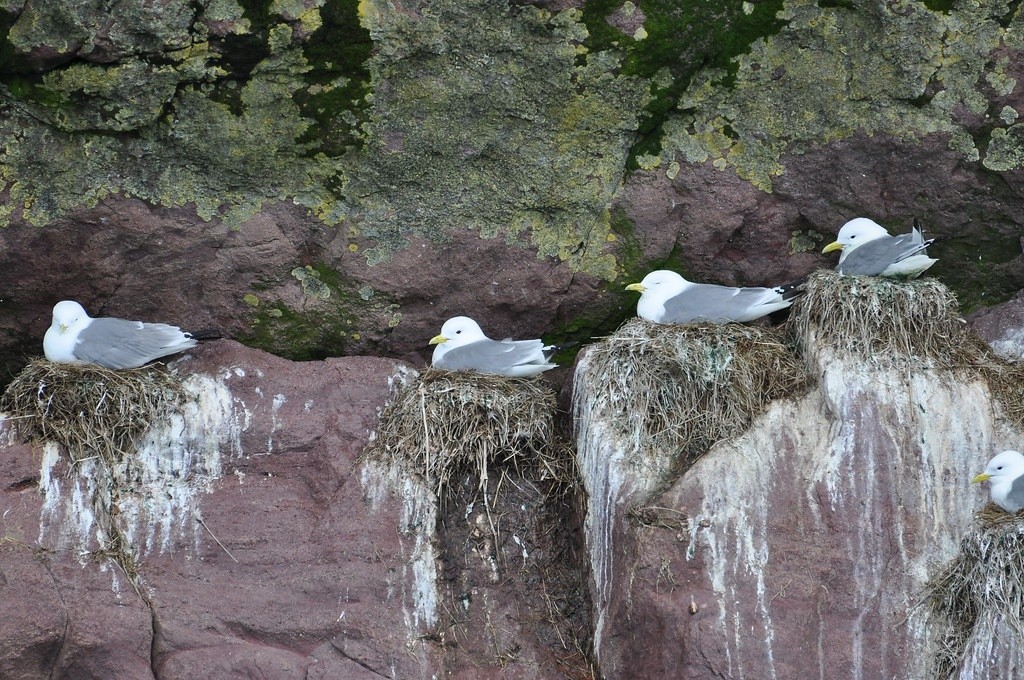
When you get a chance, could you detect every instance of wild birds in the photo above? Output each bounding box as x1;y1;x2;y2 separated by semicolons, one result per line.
41;298;205;379
425;315;562;382
969;448;1023;518
624;266;813;333
822;214;945;280
